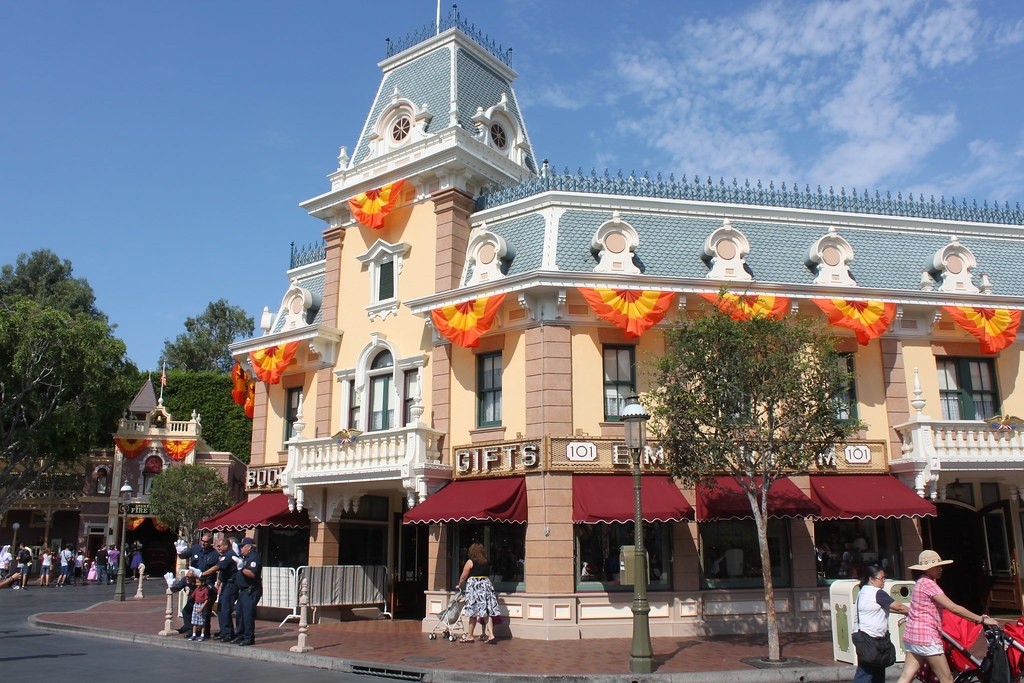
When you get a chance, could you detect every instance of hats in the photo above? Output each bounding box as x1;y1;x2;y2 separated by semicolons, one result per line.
908;550;954;571
238;537;255;546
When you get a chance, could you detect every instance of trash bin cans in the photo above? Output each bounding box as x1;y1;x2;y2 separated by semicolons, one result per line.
829;578;917;665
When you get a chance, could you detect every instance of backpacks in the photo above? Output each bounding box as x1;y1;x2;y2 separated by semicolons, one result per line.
20;550;31;564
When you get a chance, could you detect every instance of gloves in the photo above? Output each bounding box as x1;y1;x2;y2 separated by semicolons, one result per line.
164;572;176;588
11;572;21;581
232;556;244;571
188;565;202;578
174;540;188;555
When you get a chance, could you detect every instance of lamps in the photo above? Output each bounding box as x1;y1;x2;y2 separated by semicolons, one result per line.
516;432;523;439
575;428;589;436
109;528;113;535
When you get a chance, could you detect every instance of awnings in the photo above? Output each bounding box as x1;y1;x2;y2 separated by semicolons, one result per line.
809;474;938;522
572;475;696;526
403;477;528;526
198;493;310;534
696;475;821;522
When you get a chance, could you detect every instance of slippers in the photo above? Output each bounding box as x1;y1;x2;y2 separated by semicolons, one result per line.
484;639;497;644
459;638;474;643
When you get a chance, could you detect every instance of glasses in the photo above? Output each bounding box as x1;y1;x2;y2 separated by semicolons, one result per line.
873;576;885;581
200;538;209;543
217;545;221;548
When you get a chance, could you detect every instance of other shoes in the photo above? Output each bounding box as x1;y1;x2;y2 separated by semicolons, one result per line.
183;632;189;638
131;576;135;580
198;637;204;642
213;632;226;640
175;628;184;633
46;583;49;586
40;582;43;586
60;583;64;587
478;634;488;639
20;586;24;590
56;583;59;588
188;636;196;641
239;639;254;646
230;638;243;644
220;635;235;642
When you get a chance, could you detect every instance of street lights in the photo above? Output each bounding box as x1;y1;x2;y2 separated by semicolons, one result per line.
619;390;656;673
113;480;134;601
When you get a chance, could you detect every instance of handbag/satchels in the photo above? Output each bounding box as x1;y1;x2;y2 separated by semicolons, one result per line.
979;630;1014;683
67;561;75;568
50;565;54;571
851;631;896;669
837;562;849;576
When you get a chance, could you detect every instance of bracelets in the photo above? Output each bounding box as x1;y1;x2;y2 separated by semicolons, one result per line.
978;615;984;624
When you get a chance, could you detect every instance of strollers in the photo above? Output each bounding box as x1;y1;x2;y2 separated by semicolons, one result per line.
976;618;1024;683
916;609;985;683
428;587;468;642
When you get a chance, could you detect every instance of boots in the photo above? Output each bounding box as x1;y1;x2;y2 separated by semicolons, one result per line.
77;578;80;586
73;577;77;586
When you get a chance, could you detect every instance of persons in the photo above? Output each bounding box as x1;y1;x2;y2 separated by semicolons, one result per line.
164;533;263;646
456;543;501;644
0;540;143;590
817;542;865;578
851;566;910;683
896;550;999;683
